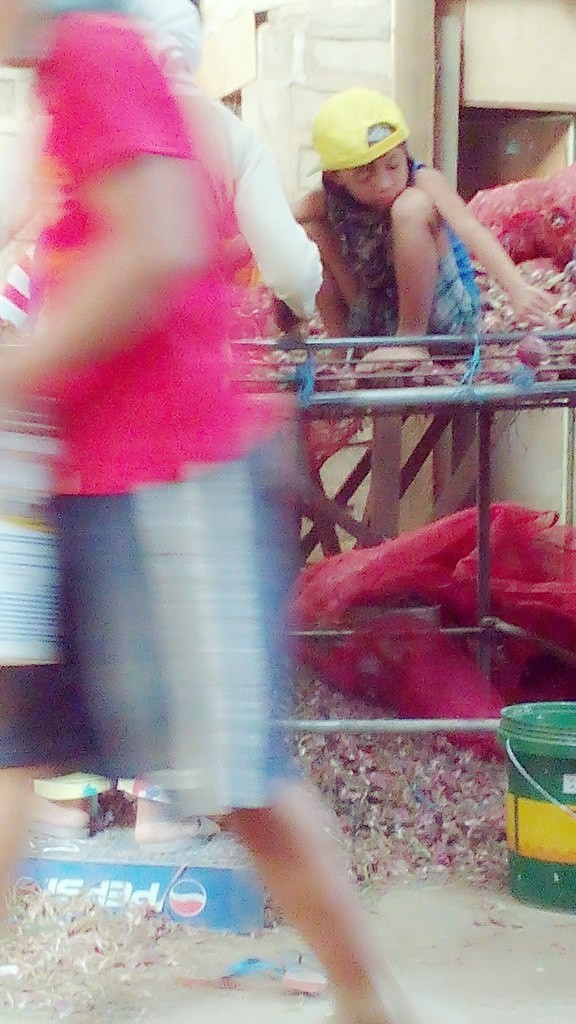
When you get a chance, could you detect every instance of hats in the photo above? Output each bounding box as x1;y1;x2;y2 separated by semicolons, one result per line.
311;88;409;170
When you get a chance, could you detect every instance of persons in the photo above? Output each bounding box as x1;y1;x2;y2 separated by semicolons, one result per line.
3;0;423;1021
291;90;557;361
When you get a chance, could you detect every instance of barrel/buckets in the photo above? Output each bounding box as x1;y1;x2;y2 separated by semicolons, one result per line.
496;702;576;916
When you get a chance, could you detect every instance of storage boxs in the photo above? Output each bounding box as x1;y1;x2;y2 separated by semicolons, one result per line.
17;825;267;930
